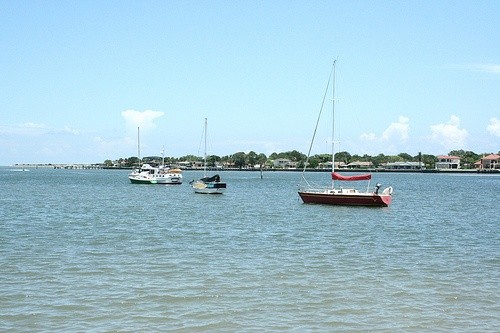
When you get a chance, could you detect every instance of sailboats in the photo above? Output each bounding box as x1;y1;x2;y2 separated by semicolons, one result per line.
128;126;183;185
191;117;227;194
298;57;395;208
